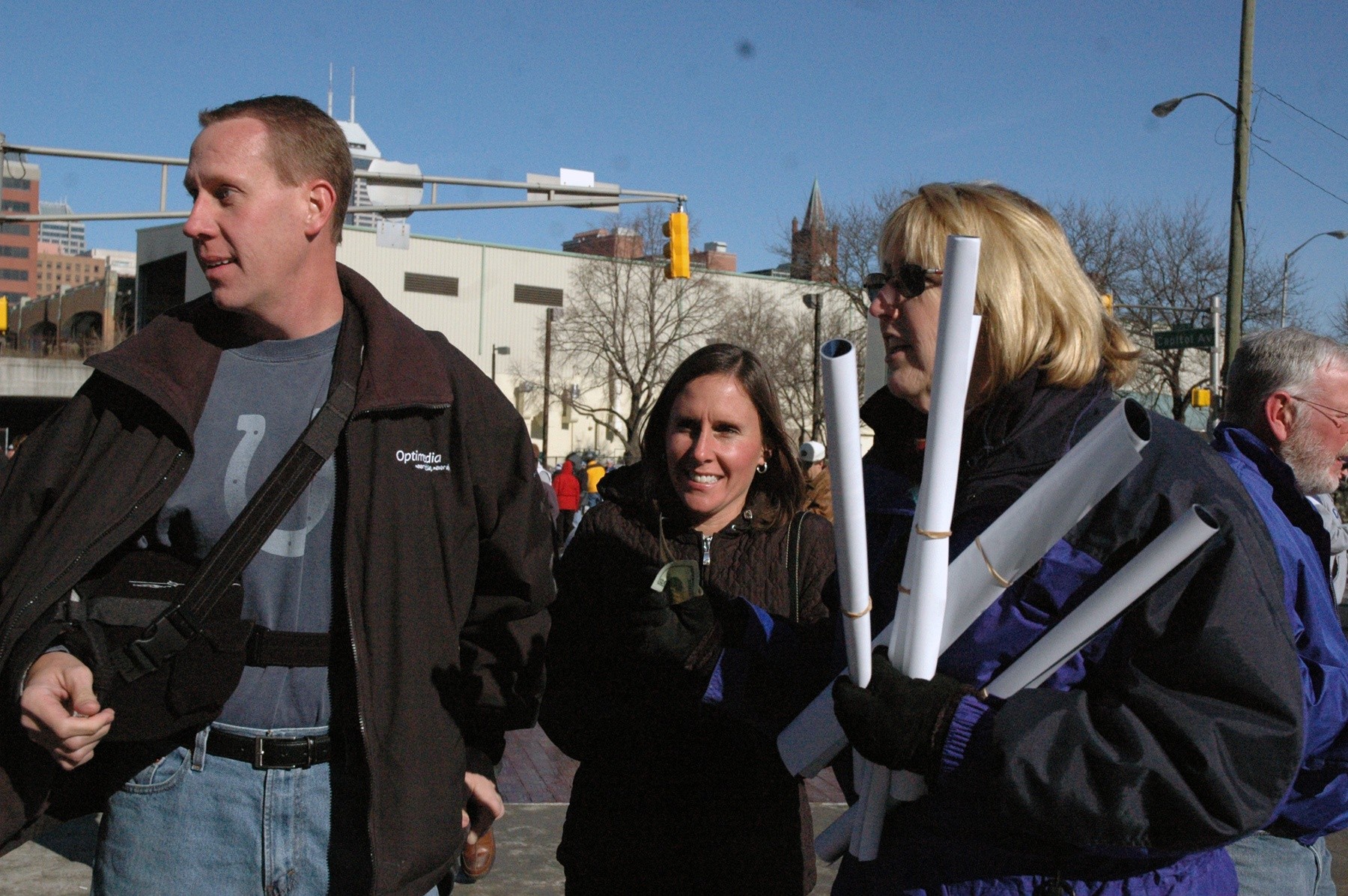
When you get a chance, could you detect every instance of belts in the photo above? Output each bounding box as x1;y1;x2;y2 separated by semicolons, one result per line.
181;725;367;771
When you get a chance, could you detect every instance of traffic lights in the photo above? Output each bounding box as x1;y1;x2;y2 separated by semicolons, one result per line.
661;212;690;280
1099;293;1114;316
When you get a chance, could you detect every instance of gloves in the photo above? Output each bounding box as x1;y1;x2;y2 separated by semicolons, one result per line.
831;645;963;776
620;565;723;677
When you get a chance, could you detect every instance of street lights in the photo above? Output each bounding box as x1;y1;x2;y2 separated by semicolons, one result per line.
1149;90;1252;395
492;343;511;382
1280;225;1348;328
802;292;823;439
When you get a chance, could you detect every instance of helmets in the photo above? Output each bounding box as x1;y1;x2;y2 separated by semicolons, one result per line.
583;451;595;462
799;441;828;463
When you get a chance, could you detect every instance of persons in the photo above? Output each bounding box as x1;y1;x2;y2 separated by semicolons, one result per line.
0;94;557;896
532;442;618;559
539;342;849;896
1205;327;1348;894
799;440;836;526
821;182;1311;895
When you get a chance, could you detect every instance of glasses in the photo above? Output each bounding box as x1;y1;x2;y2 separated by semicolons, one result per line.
863;263;943;305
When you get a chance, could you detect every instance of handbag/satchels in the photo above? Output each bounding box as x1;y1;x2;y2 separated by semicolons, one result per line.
38;544;249;823
